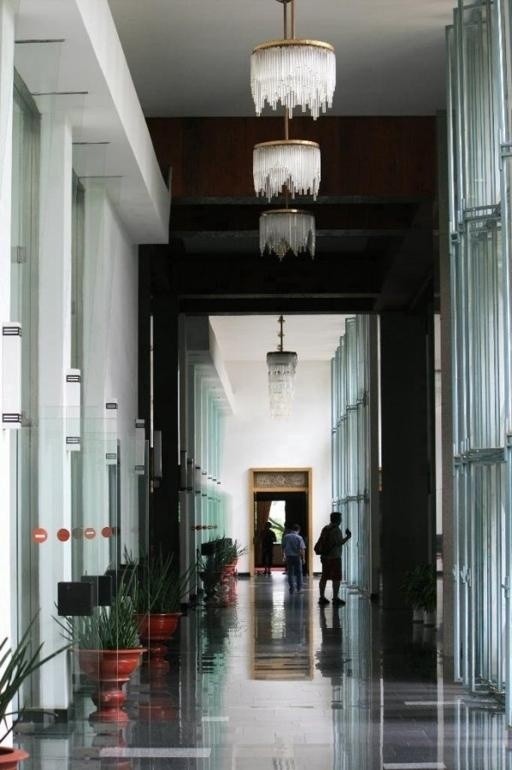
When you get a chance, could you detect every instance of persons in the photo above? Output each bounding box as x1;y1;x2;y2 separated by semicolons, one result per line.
319;512;351;606
258;522;277;575
282;524;306;595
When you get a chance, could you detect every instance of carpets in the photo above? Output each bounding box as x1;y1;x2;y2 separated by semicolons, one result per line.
255;566;288;572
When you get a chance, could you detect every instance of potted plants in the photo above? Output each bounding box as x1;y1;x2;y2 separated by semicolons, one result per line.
121;538;206;644
220;535;250;586
197;529;238;608
0;605;81;770
54;548;172;727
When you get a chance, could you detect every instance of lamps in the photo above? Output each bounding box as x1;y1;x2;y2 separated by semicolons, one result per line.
266;312;298;376
259;189;319;260
248;0;337;123
252;94;326;200
267;371;292;396
